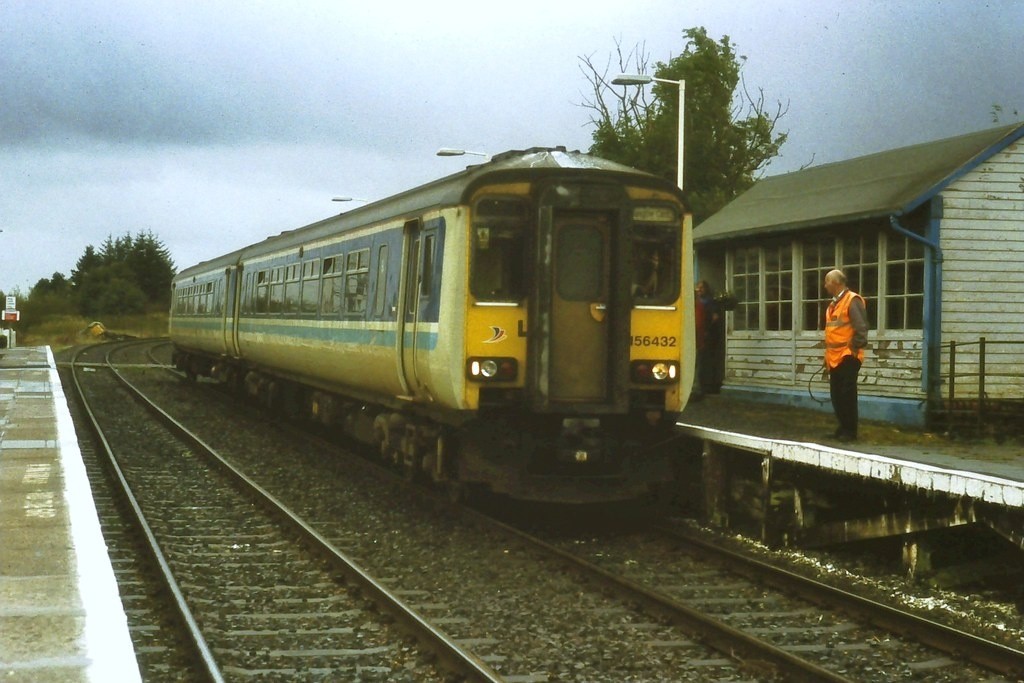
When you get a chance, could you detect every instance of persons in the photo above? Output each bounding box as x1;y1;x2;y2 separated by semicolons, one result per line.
694;281;720;394
825;270;867;442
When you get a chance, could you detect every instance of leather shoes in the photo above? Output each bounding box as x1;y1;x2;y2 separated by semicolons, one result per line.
822;432;857;442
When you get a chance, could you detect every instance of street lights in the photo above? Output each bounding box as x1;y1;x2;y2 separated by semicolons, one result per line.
610;74;686;191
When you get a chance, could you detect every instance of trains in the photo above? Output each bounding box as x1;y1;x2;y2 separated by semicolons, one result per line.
169;147;697;510
